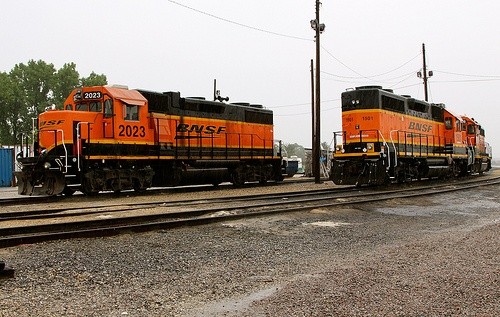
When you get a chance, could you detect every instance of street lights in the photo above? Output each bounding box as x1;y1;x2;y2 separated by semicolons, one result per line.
416;43;434;102
309;0;325;183
212;79;221;101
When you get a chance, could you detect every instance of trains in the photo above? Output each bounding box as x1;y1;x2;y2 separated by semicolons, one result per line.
329;85;493;189
13;84;284;199
